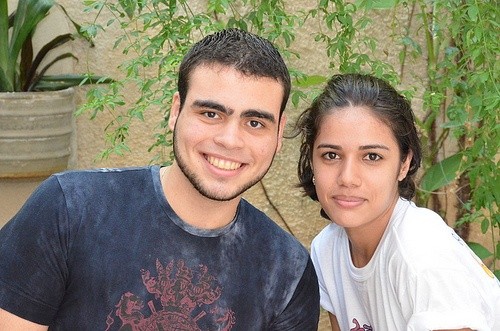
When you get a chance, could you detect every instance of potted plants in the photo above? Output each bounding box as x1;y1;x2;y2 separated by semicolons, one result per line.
0;0;124;178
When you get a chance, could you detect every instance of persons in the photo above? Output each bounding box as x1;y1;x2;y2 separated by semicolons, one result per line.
283;73;500;331
0;27;323;330
